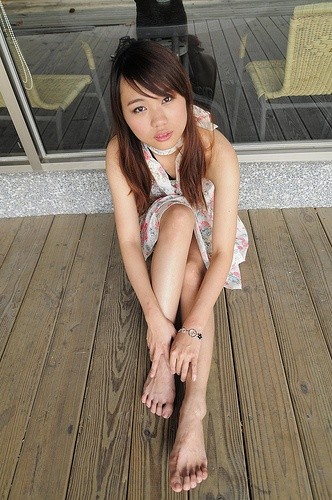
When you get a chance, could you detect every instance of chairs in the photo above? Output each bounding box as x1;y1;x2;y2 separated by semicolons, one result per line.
232;2;332;143
0;34;112;149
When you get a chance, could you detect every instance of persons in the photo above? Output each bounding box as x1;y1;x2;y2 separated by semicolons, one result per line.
136;0;218;112
106;38;249;492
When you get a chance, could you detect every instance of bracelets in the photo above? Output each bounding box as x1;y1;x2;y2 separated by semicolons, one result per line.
178;328;203;340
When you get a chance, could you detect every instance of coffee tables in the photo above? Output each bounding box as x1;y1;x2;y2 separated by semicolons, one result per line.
117;4;268;142
